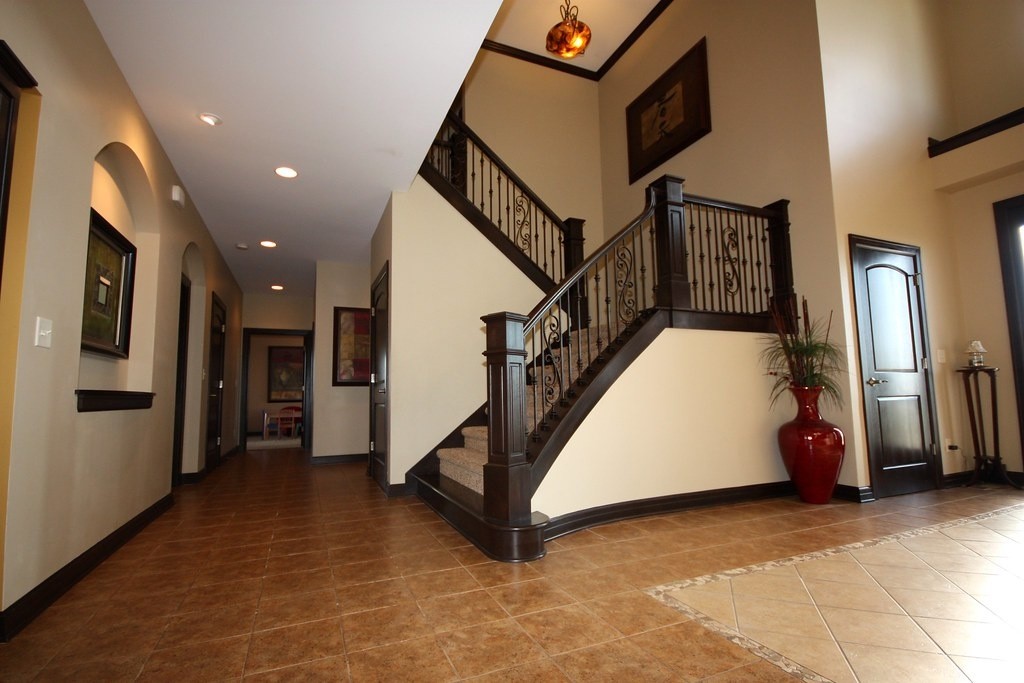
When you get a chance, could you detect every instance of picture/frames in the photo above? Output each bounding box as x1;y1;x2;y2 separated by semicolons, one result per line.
626;36;712;186
332;306;373;386
81;206;138;360
267;345;303;402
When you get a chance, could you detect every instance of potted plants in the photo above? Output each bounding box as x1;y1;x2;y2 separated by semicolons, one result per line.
752;294;847;505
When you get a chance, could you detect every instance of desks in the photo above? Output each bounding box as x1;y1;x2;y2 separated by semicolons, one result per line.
266;411;302;435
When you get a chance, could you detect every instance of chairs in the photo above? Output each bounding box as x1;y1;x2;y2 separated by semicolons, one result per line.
264;411;280;440
278;409;294;440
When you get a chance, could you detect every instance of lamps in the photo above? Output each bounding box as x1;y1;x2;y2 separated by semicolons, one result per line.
961;340;989;368
545;0;592;59
